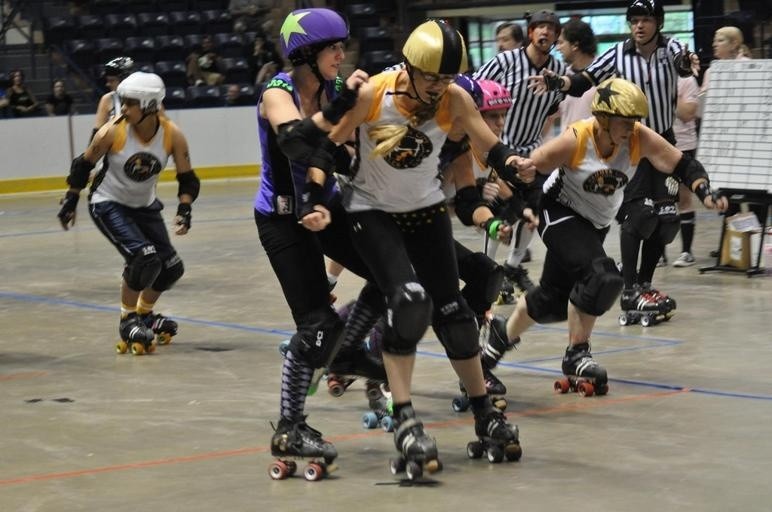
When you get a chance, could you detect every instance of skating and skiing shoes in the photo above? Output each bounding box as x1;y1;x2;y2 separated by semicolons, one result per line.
553;342;609;397
494;277;516;306
324;338;388;398
267;414;339;481
466;395;522;465
641;282;677;321
478;310;520;371
362;378;397;433
389;404;444;481
617;281;665;327
453;368;508;420
503;260;536;298
116;312;157;355
136;310;177;346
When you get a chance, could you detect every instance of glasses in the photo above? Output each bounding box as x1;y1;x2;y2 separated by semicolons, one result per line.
120;98;139;107
420;71;457;84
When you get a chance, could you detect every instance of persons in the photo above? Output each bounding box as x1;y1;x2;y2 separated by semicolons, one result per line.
9;70;38;115
278;73;512;431
458;11;571;305
472;77;728;401
551;20;605;148
199;35;226;82
296;15;536;485
46;82;77;115
87;59;132;199
495;21;522;53
252;10;390;483
656;68;699;268
527;3;692;328
314;77;513;425
60;71;199;357
694;25;748;64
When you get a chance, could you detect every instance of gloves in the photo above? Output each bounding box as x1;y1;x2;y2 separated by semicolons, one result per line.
695;183;726;207
475;177;499;203
322;77;360;126
502;195;540;231
175;203;192;230
673;45;700;79
486;141;526;187
57;192;80;230
298;180;325;221
542;72;565;92
480;216;513;246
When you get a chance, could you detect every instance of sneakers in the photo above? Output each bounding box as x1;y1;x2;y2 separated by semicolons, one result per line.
672;252;696;267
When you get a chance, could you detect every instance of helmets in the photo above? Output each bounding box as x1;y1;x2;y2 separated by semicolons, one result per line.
114;72;166;114
401;19;470;75
455;73;474;94
279;7;349;59
626;1;664;20
470;80;512;111
527;9;561;35
590;78;650;118
106;57;134;75
